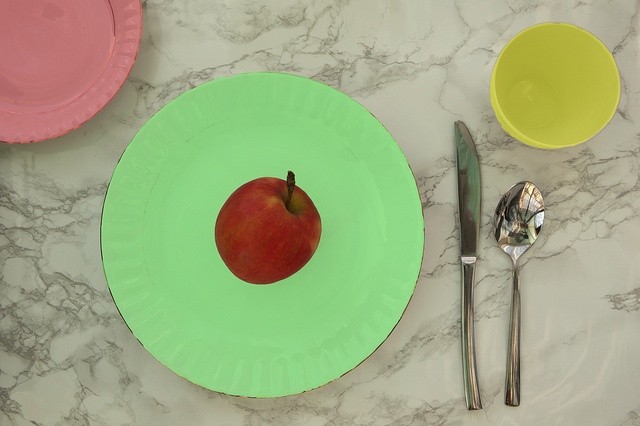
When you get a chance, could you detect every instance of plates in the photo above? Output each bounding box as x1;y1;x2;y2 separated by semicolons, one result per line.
1;0;142;144
98;71;425;399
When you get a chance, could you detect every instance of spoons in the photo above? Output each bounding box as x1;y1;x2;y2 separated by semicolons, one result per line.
490;180;546;408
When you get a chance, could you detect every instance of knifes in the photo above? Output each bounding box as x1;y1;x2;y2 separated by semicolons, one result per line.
452;117;484;410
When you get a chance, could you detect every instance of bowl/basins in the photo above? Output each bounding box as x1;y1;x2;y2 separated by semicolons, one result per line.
489;22;622;150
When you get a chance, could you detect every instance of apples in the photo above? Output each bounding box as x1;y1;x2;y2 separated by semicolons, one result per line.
215;171;322;284
